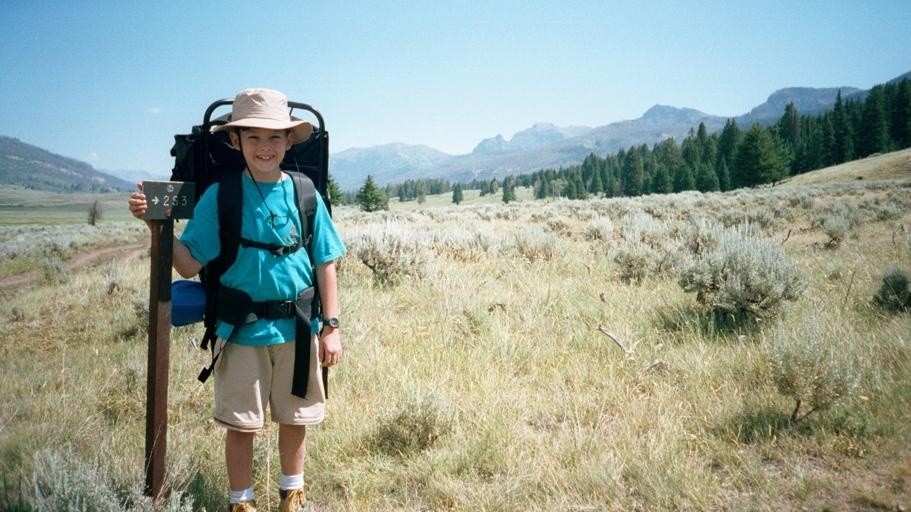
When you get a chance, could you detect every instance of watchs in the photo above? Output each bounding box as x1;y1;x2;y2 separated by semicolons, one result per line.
324;317;339;328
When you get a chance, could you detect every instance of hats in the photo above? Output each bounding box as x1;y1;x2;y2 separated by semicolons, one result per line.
215;90;314;144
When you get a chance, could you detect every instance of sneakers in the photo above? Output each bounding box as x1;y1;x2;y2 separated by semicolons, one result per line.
228;499;257;511
279;488;304;512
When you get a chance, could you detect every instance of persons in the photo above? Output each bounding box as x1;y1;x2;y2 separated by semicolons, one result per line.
128;88;347;512
